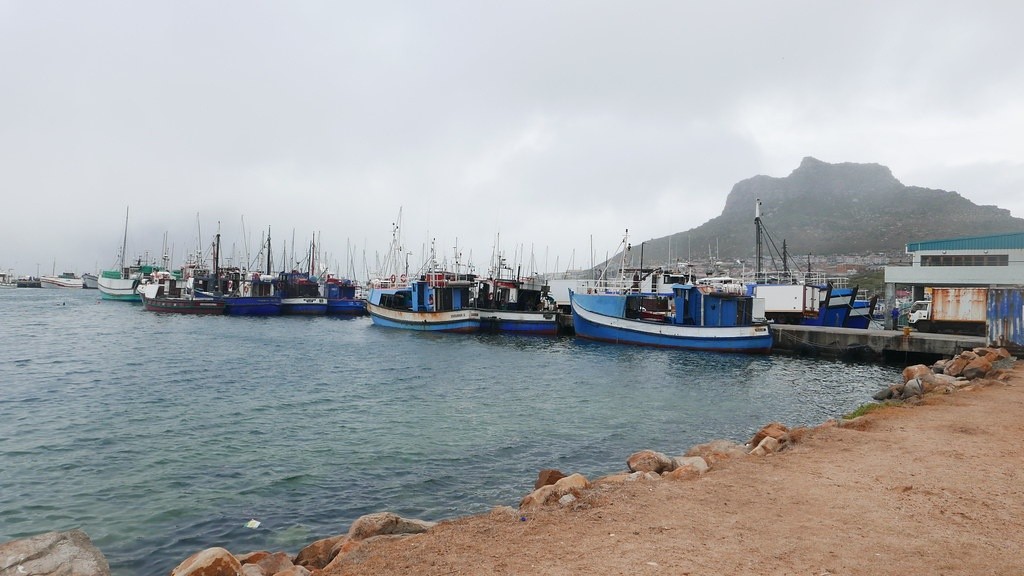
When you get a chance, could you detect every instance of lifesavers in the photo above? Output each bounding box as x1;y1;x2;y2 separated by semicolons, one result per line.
489;291;495;301
428;295;433;304
228;282;232;288
390;274;396;283
401;274;406;283
244;286;248;292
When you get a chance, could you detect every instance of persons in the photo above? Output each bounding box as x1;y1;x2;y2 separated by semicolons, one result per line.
891;306;900;331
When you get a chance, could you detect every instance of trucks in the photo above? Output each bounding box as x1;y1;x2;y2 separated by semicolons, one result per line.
907;286;988;337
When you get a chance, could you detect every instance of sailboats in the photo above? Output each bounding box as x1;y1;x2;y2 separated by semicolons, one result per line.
567;228;775;355
474;231;564;337
366;236;481;335
0;196;368;316
744;196;820;325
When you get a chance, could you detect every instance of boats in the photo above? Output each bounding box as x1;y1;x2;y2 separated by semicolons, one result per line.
806;250;860;328
781;239;835;325
843;294;880;328
